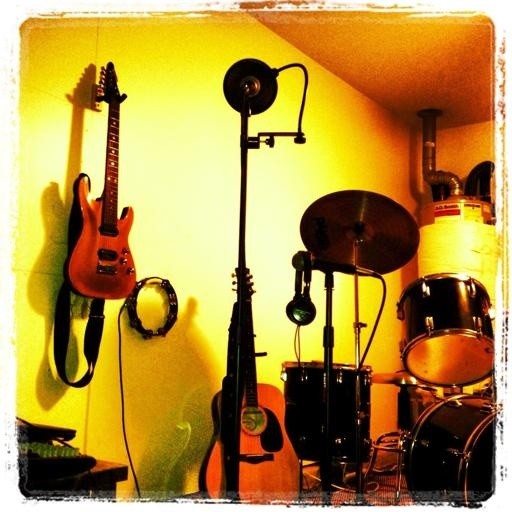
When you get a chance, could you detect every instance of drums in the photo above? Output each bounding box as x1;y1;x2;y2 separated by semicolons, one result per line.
404;392;495;503
398;273;494;387
281;359;372;465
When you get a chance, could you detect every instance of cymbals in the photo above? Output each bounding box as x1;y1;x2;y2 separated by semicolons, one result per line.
369;371;462;392
300;190;420;274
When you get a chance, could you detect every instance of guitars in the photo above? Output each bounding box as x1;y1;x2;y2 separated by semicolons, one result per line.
66;62;136;299
199;266;301;502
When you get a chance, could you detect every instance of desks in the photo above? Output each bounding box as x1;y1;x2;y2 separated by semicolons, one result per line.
17;450;132;502
